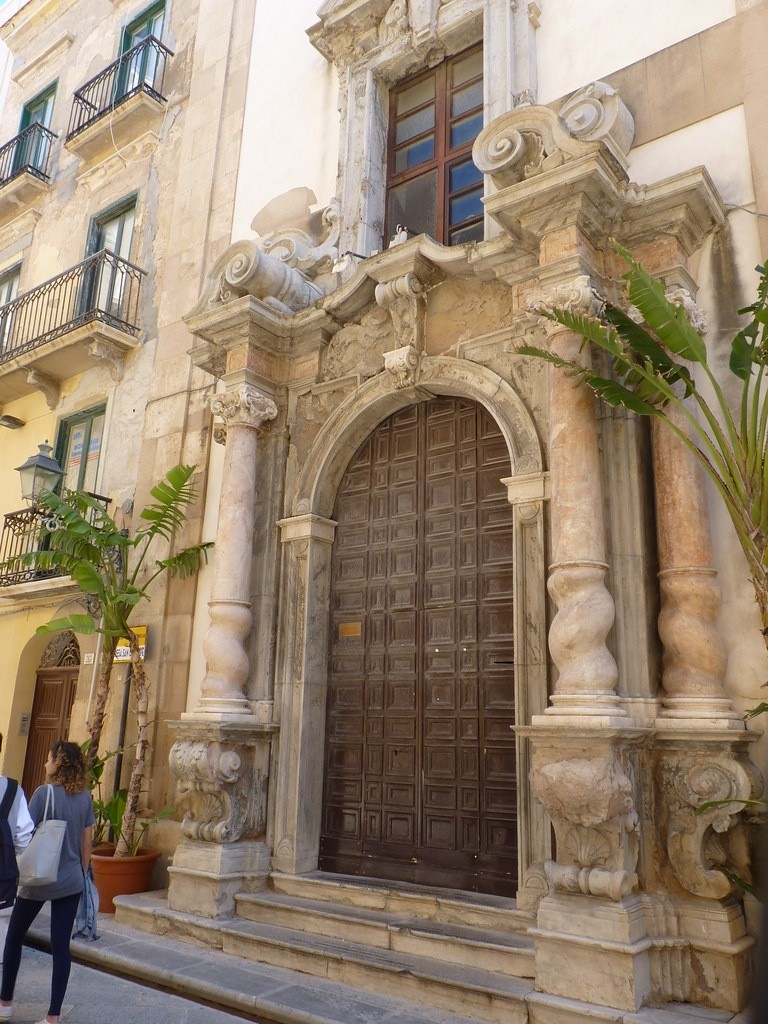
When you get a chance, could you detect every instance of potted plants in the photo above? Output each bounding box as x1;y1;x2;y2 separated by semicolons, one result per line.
1;464;214;914
80;737;135;848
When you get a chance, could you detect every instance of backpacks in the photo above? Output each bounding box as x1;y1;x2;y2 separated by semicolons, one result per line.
0;777;20;910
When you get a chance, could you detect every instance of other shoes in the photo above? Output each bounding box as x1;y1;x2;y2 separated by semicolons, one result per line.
35;1017;50;1024
0;1006;13;1022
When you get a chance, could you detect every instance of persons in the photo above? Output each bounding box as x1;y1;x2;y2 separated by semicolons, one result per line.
0;740;95;1024
0;733;35;910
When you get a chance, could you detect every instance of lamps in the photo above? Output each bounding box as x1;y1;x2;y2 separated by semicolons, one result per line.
0;415;26;429
14;438;128;538
332;251;368;273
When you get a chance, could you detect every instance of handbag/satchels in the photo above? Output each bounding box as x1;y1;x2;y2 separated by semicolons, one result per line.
16;784;67;886
